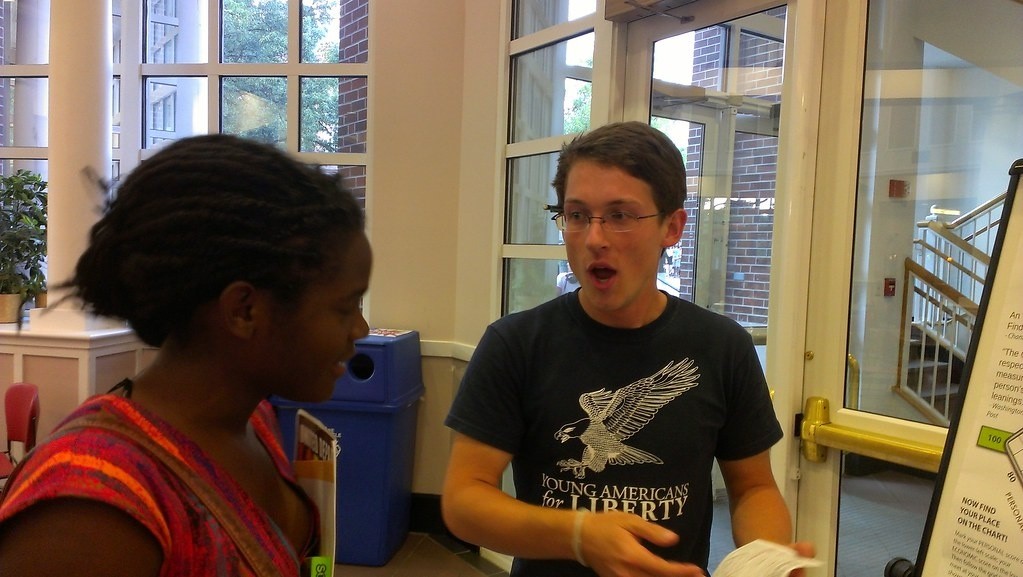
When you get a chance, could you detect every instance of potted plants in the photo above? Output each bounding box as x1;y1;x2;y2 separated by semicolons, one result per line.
30;194;47;308
0;169;51;324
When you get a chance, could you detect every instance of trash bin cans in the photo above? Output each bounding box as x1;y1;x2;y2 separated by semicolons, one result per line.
267;327;425;568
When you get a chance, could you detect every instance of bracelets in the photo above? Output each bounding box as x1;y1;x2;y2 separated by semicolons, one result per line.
571;508;591;566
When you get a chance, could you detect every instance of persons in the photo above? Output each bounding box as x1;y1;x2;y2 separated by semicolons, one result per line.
0;135;372;577
442;121;816;577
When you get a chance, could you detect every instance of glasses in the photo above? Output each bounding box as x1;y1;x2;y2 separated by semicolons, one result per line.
552;209;664;233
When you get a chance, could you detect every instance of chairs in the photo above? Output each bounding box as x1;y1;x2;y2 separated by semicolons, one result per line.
0;382;42;483
